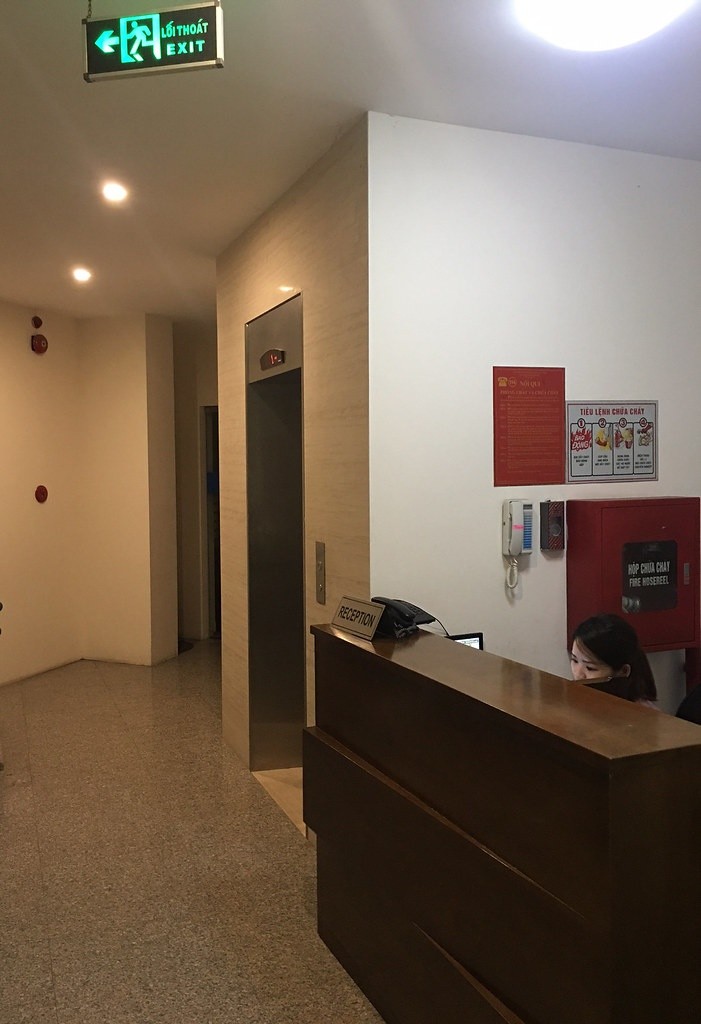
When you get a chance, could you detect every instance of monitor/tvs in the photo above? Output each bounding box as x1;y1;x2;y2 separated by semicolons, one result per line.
445;632;484;651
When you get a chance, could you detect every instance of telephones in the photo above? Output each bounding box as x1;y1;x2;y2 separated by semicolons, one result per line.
370;597;436;639
503;499;533;556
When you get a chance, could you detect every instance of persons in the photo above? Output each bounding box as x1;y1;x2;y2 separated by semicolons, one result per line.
571;613;663;714
